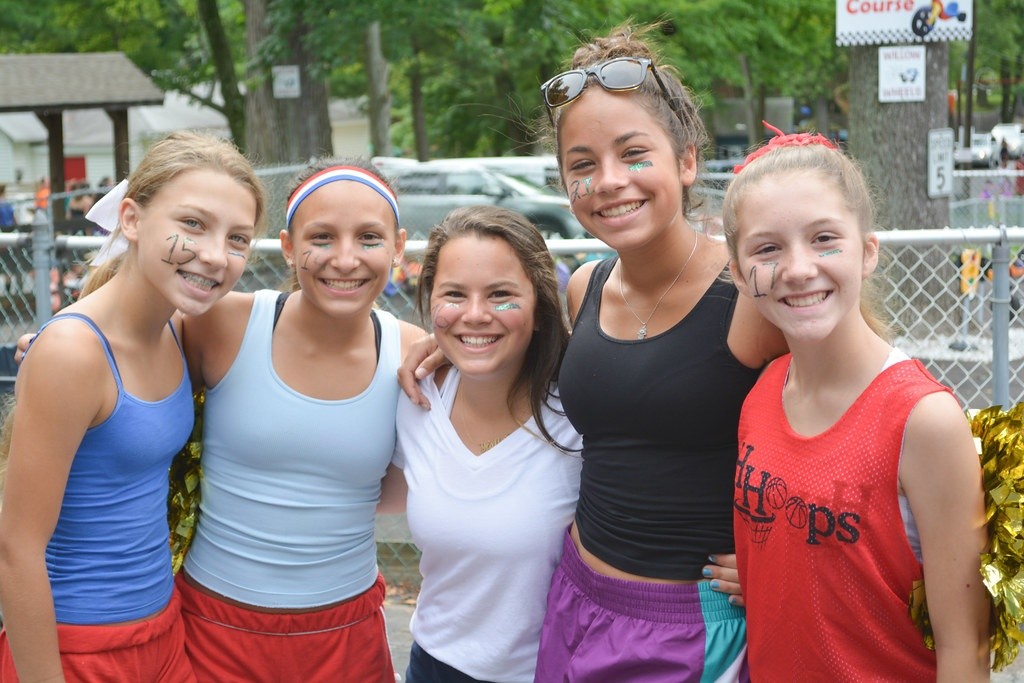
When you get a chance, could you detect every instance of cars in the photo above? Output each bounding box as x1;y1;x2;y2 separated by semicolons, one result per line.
953;133;998;169
992;123;1024;157
245;144;751;240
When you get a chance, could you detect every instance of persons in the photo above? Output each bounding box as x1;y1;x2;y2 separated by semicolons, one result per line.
716;120;995;682
376;204;745;683
13;150;431;683
999;137;1010;170
397;10;791;683
0;129;272;683
0;174;113;235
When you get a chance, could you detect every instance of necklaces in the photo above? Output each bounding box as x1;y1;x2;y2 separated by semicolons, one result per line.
618;229;698;340
460;382;535;452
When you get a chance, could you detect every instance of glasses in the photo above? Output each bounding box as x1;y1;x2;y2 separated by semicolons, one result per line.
540;55;676;131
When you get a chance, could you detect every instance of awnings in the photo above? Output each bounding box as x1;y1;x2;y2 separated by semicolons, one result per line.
0;51;165;109
